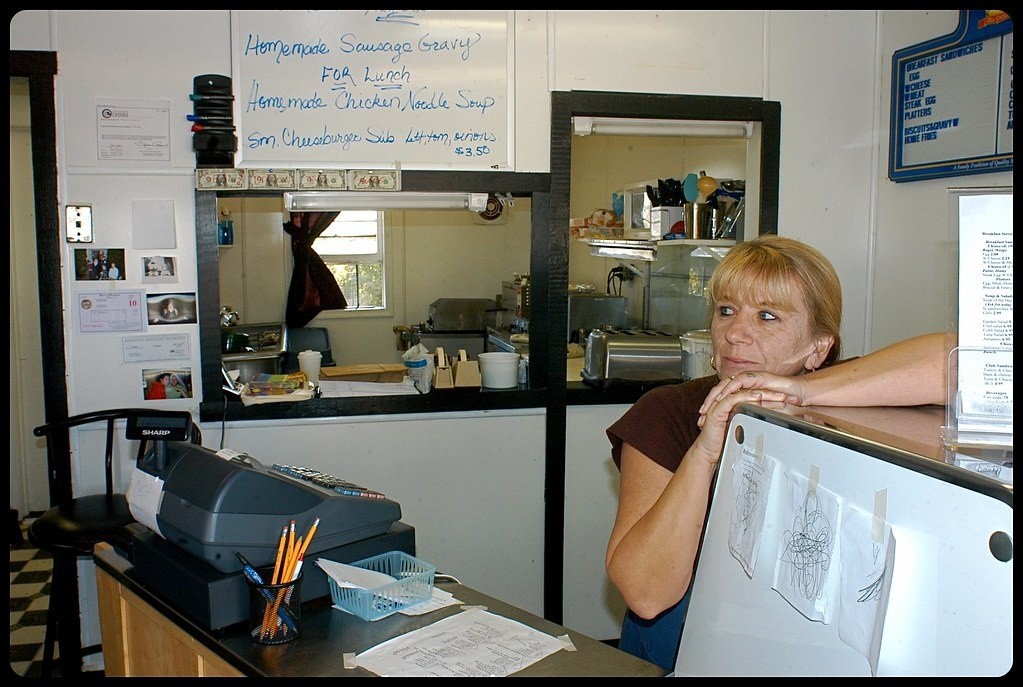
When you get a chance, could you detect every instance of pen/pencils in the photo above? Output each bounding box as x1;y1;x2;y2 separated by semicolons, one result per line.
236;516;322;645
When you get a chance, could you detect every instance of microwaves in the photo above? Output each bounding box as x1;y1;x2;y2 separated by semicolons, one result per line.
623;174;734;240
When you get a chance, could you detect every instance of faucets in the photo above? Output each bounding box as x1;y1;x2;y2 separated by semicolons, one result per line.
222;310;241;321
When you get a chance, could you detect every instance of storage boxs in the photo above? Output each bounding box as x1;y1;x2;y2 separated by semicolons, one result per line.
685;200;725;239
479;351;521;388
244;567;304;643
650;208;686;242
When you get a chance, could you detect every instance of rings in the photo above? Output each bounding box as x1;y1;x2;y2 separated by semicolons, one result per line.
726;375;735;380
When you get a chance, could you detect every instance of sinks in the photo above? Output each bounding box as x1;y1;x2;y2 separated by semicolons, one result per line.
220;321;288;387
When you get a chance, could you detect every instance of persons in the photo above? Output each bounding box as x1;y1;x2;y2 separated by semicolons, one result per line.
79;250;121;279
147;373;169;400
161;300;178;320
604;230;961;674
164;373;190;400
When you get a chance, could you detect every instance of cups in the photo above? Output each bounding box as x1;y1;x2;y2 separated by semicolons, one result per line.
297;349;323;385
684;202;712;240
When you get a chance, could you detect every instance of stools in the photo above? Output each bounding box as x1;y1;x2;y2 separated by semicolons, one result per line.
26;410;200;682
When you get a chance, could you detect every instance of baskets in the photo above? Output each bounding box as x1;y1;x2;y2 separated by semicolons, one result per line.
329;551;436;621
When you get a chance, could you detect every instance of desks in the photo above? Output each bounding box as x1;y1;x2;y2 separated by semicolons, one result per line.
91;519;667;678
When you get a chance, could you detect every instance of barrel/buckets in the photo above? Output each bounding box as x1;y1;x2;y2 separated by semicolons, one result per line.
477;352;521;389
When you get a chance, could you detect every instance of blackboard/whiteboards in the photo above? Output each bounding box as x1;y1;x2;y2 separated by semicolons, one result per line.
230;10;516;172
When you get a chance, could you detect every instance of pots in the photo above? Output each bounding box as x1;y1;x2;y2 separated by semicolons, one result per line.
224;356;280;384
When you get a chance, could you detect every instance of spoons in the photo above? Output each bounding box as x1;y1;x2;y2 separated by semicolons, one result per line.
696;176;717;203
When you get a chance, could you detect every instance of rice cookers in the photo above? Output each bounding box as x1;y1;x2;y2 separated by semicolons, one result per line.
678;328;719;382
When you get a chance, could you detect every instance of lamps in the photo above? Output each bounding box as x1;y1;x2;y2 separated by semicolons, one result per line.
572;117;754;139
283;191;488;213
